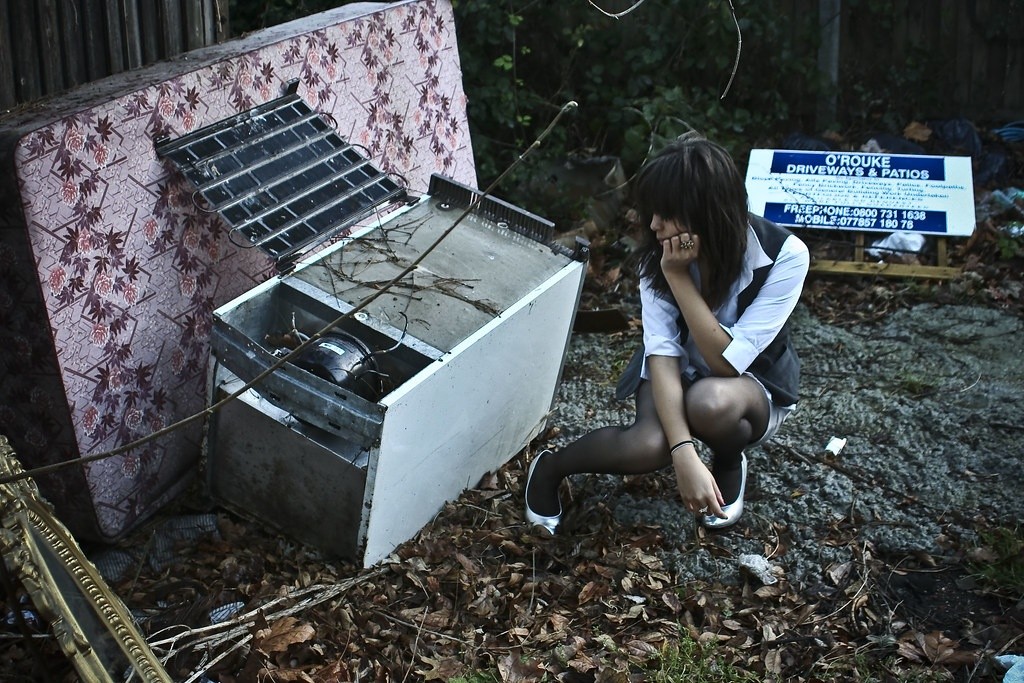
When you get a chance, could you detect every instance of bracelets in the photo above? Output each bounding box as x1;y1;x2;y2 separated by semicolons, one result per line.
670;440;695;455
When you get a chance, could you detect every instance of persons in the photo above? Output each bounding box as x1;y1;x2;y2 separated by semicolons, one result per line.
525;137;812;536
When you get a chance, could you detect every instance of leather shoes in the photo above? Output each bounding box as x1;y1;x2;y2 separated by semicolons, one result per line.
525;450;563;535
702;451;747;528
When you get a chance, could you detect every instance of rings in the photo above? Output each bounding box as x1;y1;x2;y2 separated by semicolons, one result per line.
679;240;695;250
